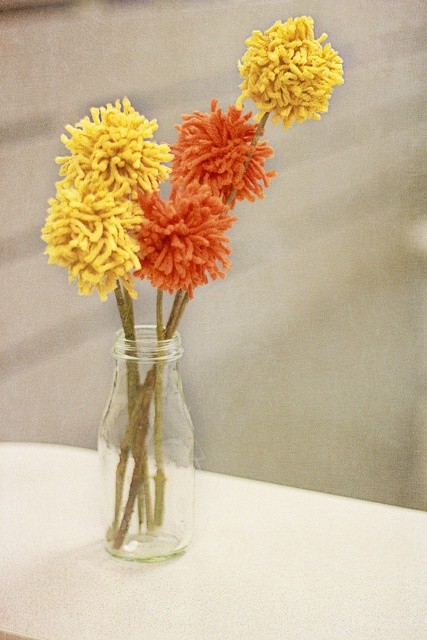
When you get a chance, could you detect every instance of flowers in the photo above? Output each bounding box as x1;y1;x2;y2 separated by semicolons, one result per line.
40;14;345;549
